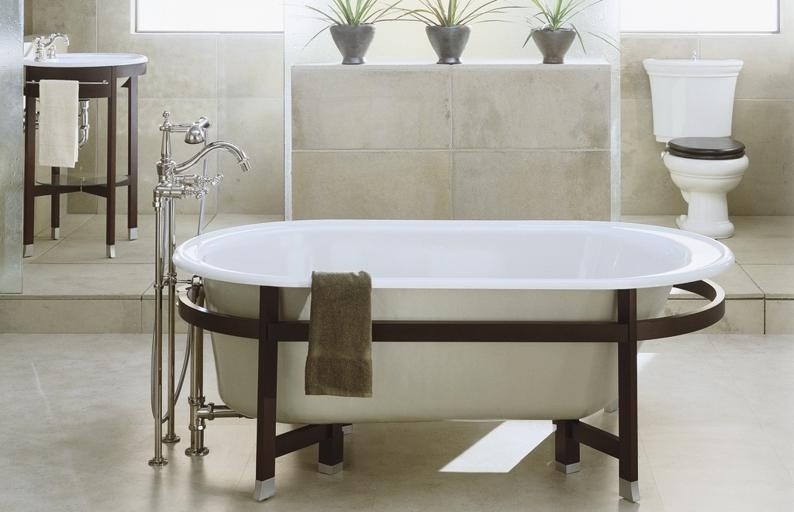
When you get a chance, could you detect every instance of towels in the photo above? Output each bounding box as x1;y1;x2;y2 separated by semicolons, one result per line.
300;268;375;399
36;76;80;169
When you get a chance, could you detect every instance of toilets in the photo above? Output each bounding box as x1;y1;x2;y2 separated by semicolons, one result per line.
643;58;748;238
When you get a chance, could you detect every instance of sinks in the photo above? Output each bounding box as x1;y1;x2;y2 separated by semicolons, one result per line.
26;53;148;69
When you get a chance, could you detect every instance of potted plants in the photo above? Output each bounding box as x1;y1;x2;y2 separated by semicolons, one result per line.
296;0;427;67
518;0;629;67
385;0;524;65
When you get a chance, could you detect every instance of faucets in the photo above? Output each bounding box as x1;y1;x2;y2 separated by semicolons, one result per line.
45;33;70;49
176;140;251;174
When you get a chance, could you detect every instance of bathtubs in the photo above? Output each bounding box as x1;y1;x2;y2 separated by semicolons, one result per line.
171;220;734;425
